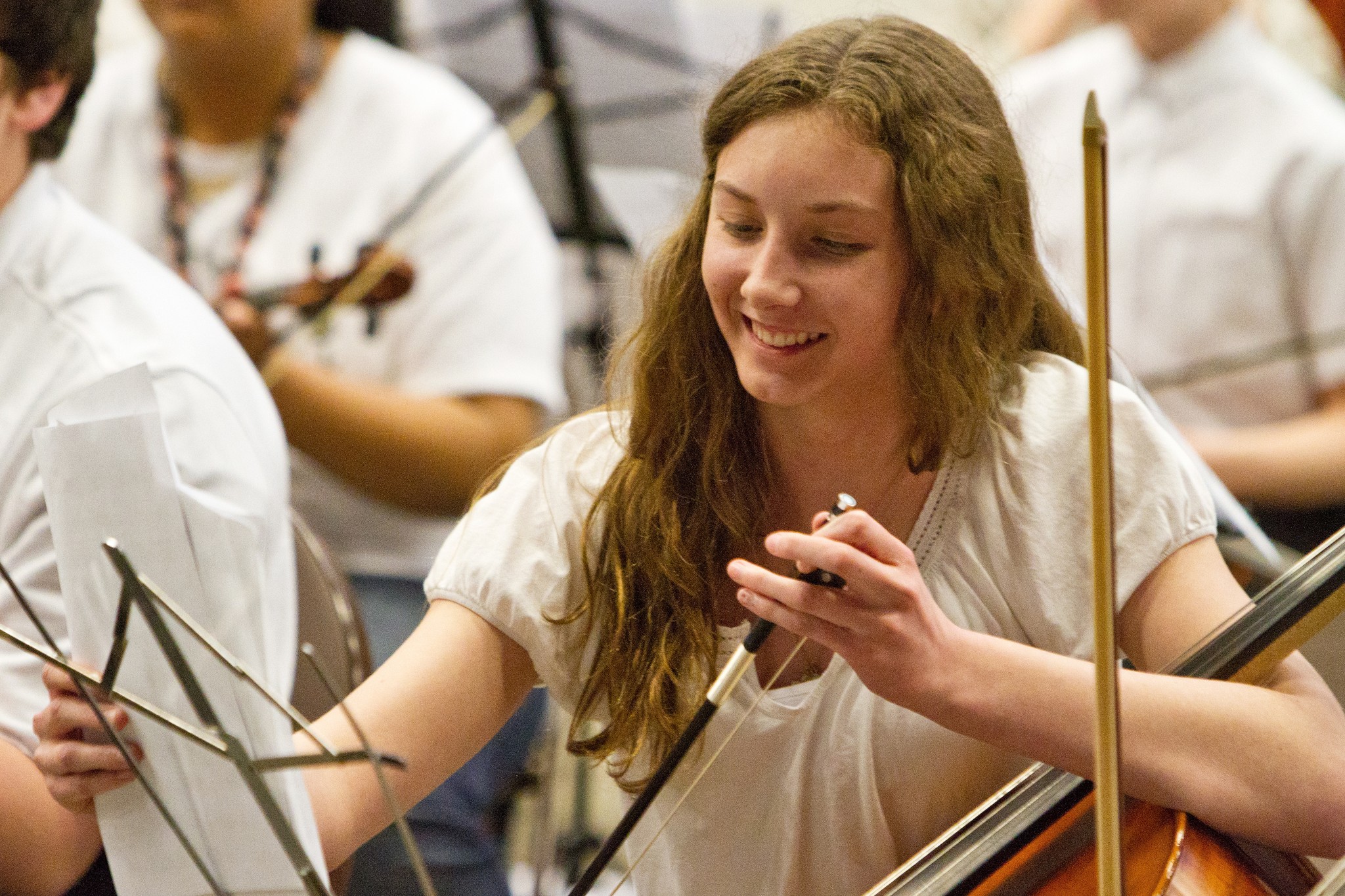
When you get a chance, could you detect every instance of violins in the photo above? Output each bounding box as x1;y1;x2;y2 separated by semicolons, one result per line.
198;236;420;362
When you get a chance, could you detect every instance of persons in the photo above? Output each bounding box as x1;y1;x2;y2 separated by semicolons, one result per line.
987;0;1345;502
0;0;298;896
35;15;1345;896
34;0;569;895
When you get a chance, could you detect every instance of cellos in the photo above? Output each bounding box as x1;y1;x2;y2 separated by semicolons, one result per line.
866;522;1342;894
